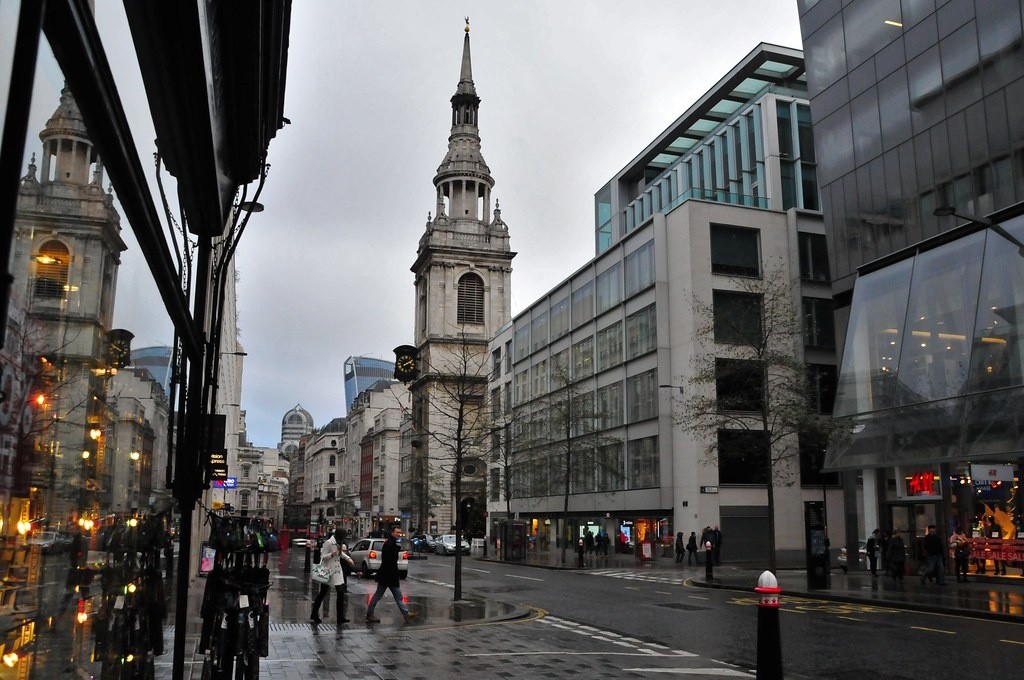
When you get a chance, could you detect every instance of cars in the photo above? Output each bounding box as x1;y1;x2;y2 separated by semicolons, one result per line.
408;534;443;553
837;540;913;573
435;534;470;555
349;538;409;580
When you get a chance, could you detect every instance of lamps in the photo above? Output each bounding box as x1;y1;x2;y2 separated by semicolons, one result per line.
933;208;991;226
659;385;684;393
988;224;1024;257
233;202;264;212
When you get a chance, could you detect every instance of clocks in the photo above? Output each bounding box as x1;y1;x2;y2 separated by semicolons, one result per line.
393;345;420;386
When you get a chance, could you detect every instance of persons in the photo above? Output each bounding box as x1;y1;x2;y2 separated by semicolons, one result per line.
309;528;354;625
699;525;722;565
584;531;595;557
950;526;969;583
919;525;948;587
365;525;419;623
675;532;685;566
595;532;610;558
688;532;700;567
886;531;905;582
61;521;89;620
621;530;629;542
971;515;1006;575
866;529;891;577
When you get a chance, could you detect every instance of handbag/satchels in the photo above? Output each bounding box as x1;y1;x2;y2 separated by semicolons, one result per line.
954;545;969;558
311;563;334;583
875;551;880;557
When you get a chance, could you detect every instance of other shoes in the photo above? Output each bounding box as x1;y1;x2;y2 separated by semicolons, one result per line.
404;612;417;621
337;619;351;624
366;616;380;623
310;616;322;623
